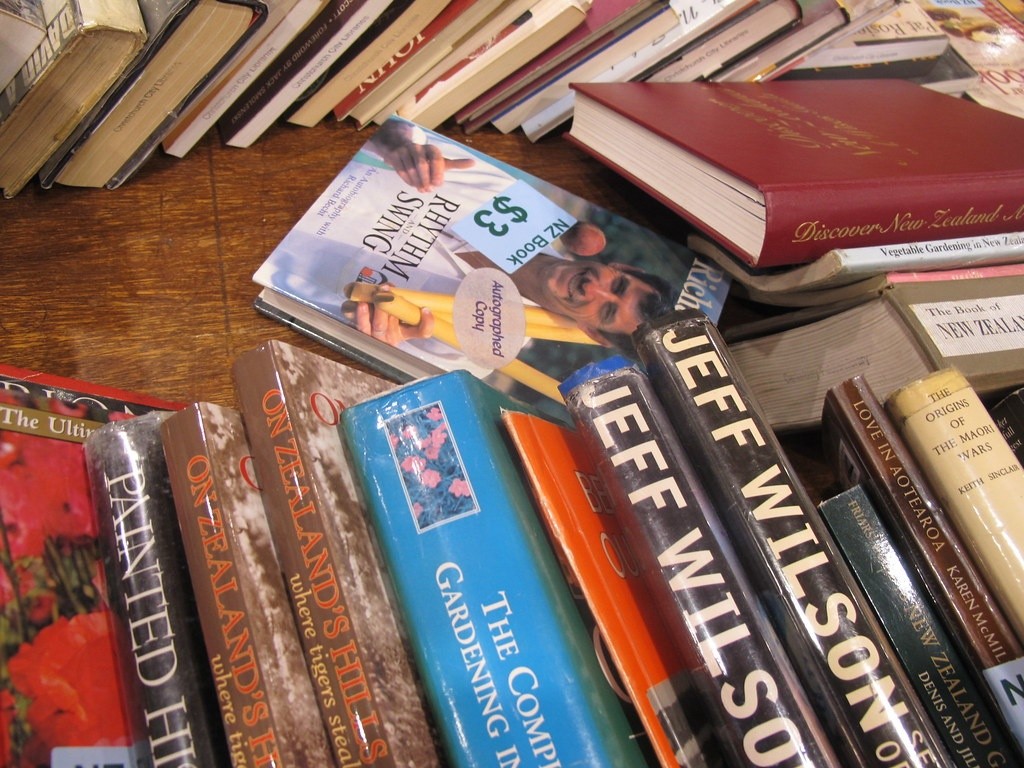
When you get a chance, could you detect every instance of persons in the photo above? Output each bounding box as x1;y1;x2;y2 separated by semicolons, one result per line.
257;112;694;358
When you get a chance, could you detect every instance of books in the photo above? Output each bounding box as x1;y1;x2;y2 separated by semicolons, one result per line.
564;73;1024;272
0;0;1024;768
337;369;665;768
